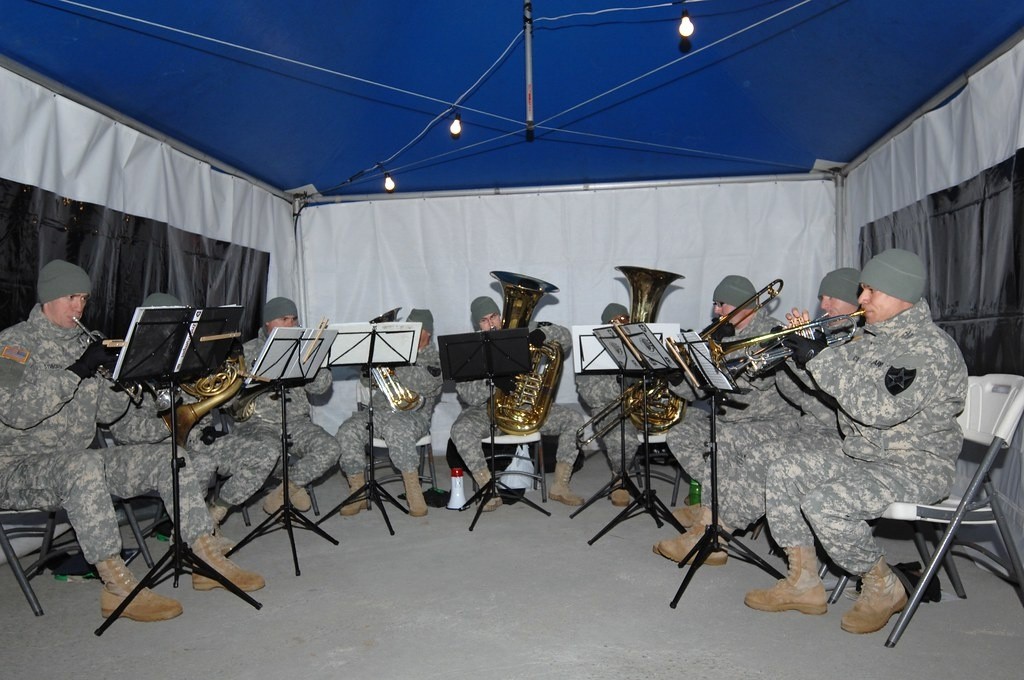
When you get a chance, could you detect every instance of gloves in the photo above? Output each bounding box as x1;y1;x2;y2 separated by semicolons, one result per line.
529;329;545;348
65;335;117;380
492;374;516;395
768;325;823;368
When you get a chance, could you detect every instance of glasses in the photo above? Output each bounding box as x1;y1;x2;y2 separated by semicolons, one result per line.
608;315;627;324
713;302;724;307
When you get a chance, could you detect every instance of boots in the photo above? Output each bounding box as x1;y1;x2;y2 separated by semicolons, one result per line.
471;467;503;511
340;473;370;515
95;554;183;622
400;470;428;517
653;502;735;565
548;461;584;505
191;534;266;592
744;545;828;615
611;470;630;505
262;477;311;523
841;556;907;635
206;494;228;537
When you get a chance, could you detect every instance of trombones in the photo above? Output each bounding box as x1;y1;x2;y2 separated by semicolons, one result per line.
576;278;784;446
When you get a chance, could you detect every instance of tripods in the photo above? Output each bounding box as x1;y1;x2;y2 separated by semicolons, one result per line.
224;329;339;576
321;326;415;535
94;308;264;636
568;325;787;608
445;330;552;531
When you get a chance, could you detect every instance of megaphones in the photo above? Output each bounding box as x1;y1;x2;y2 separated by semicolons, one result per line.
446;467;470;509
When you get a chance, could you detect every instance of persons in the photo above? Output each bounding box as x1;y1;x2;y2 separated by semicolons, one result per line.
0;260;265;622
575;304;711;505
240;298;342;515
653;268;860;565
451;297;585;508
667;275;786;526
745;248;968;637
336;307;444;515
96;293;279;557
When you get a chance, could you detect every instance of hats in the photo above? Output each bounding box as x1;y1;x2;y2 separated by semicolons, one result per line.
262;297;296;323
818;267;861;306
142;293;181;307
470;296;500;325
406;308;433;334
860;248;925;304
601;303;628;323
712;275;756;308
36;259;92;304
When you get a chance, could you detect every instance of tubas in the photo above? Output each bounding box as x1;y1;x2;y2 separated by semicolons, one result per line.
162;354;245;449
360;306;425;414
486;269;564;436
614;266;687;433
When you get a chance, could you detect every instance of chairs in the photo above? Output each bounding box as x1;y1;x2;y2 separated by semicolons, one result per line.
751;374;1024;649
475;432;546;504
0;405;320;616
357;378;437;511
609;432;682;506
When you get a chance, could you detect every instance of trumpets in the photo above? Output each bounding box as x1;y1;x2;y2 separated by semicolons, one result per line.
706;308;866;362
